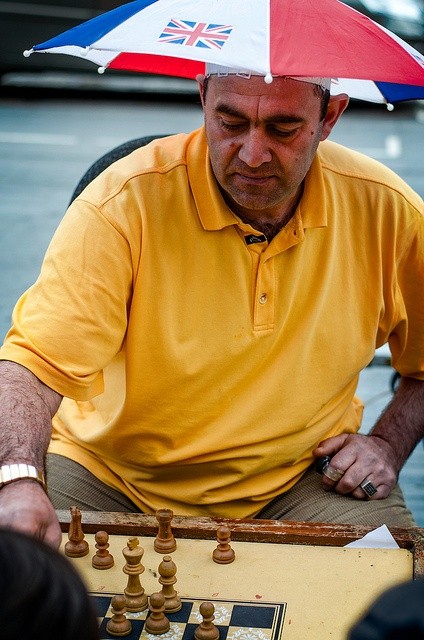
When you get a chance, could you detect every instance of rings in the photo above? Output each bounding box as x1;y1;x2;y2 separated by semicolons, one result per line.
359;481;379;499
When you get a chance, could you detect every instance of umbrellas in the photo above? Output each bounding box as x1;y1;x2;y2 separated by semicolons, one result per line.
25;1;424;110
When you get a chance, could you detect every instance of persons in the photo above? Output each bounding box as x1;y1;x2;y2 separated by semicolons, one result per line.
0;61;424;554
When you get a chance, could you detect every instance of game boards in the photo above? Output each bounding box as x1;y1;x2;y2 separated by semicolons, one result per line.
53;533;414;640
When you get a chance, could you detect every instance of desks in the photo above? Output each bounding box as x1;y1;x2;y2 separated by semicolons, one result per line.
51;509;424;640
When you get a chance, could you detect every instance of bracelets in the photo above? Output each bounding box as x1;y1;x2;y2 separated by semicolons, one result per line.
0;464;47;487
